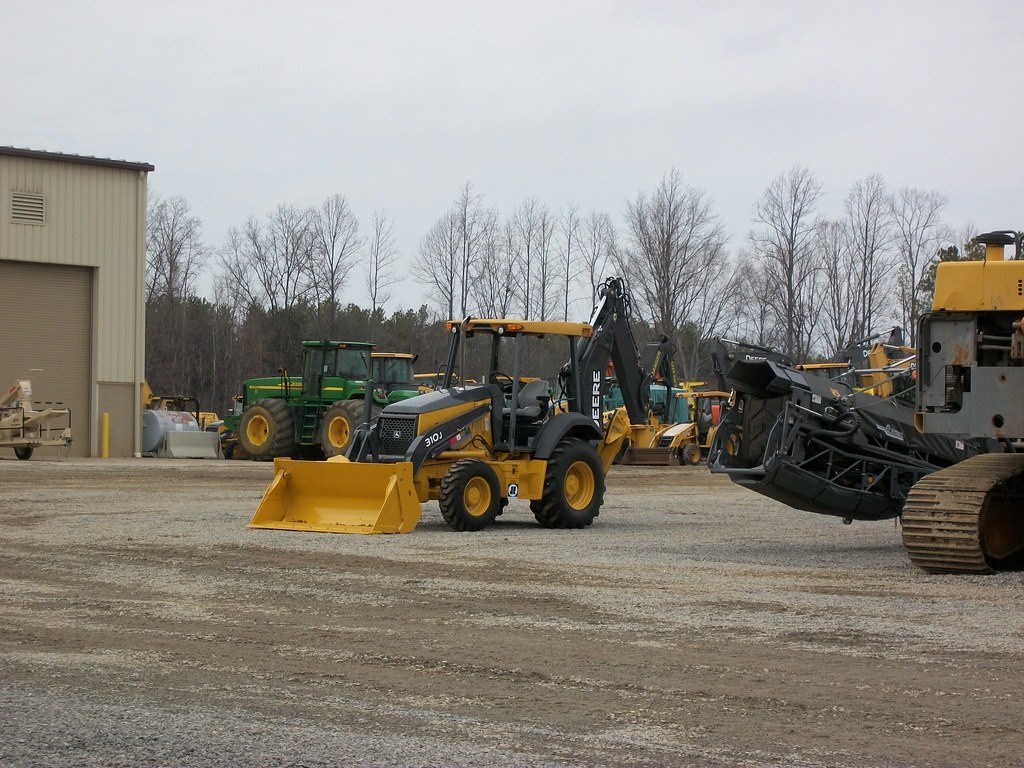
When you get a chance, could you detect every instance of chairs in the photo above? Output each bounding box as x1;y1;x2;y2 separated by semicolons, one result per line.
502;380;550;418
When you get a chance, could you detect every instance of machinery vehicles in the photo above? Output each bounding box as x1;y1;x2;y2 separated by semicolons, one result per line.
225;339;429;463
141;380;238;452
900;231;1024;574
360;352;541;397
553;277;982;526
244;316;607;535
